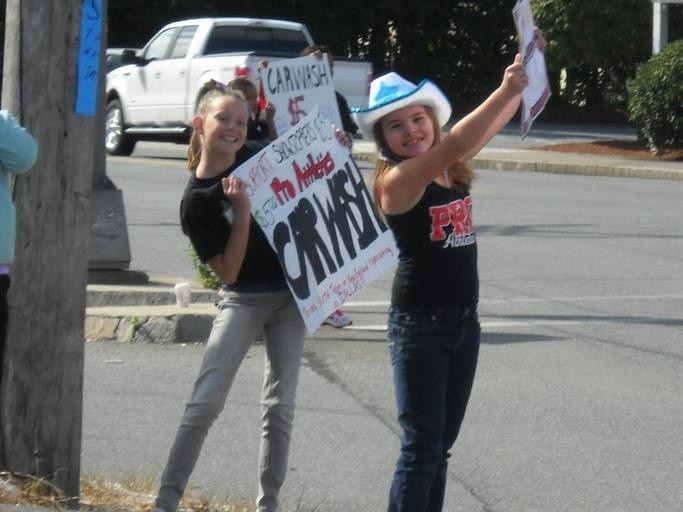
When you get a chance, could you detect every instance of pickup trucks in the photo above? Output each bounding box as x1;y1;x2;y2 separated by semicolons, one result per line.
107;17;370;155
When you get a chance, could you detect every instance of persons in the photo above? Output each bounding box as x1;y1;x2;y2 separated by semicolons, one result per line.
0;109;39;474
228;45;359;327
151;80;353;512
349;26;551;512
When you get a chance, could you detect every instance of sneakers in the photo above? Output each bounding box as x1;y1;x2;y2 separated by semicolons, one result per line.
321;309;354;330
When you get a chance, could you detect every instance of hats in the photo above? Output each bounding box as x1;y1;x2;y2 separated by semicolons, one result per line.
351;70;454;142
